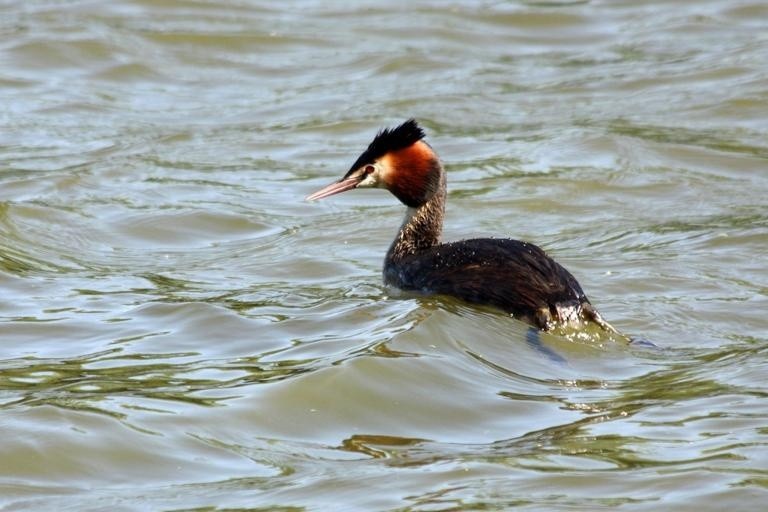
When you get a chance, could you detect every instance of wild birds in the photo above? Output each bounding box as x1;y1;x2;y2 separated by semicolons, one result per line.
305;116;623;343
